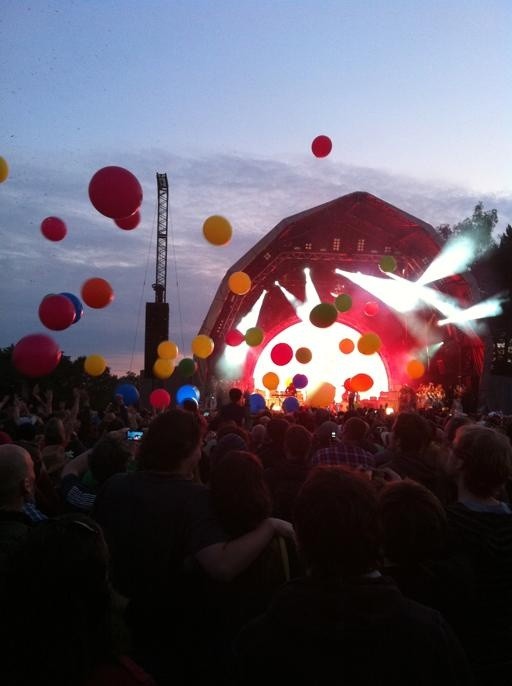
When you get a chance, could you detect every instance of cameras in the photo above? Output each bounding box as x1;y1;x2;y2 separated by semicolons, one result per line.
126;429;144;441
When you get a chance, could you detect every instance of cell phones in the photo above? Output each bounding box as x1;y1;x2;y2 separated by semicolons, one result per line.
330;431;336;441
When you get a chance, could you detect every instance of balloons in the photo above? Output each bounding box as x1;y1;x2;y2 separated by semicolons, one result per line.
406;358;425;380
